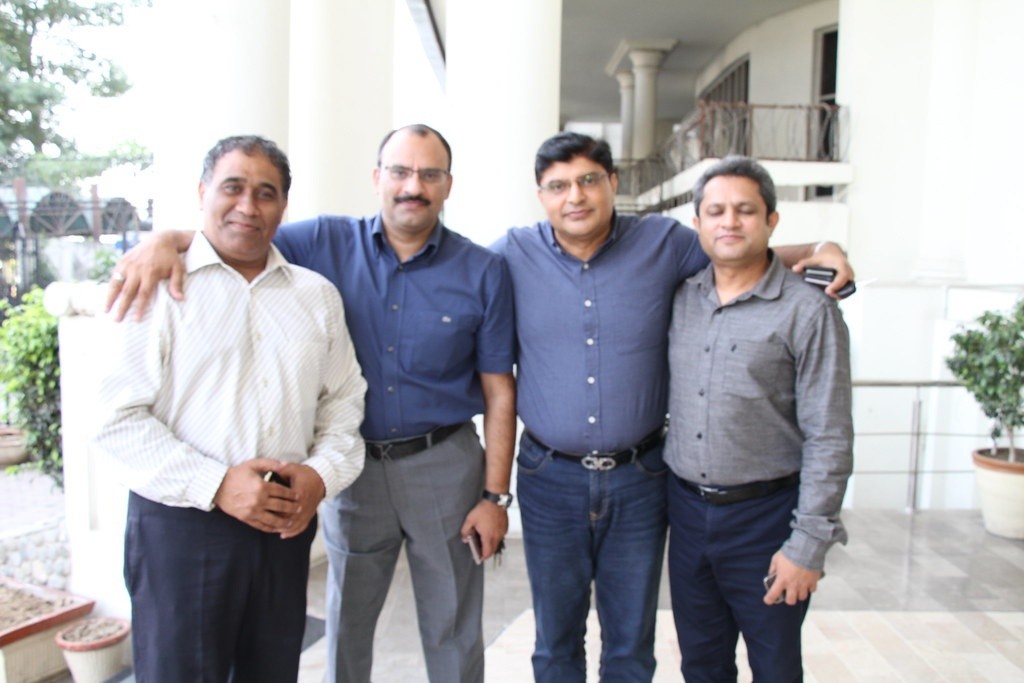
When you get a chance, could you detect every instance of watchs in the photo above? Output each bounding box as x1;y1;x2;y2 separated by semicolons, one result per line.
482;490;513;509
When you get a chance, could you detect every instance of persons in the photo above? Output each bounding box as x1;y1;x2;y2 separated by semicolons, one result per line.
103;122;855;683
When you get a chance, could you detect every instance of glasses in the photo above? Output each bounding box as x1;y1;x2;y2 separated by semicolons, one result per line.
382;165;449;185
540;173;609;194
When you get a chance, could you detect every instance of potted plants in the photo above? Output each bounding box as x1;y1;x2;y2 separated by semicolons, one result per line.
945;301;1023;538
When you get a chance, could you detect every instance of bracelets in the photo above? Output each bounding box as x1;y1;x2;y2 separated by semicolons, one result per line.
813;240;829;258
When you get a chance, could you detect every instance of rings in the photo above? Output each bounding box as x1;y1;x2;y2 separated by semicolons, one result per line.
113;273;126;283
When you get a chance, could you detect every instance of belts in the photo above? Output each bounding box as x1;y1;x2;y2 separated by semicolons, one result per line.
524;426;666;470
681;474;800;507
365;422;463;461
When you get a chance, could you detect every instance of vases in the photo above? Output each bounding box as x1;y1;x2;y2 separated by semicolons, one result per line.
0;429;29;466
54;617;131;683
